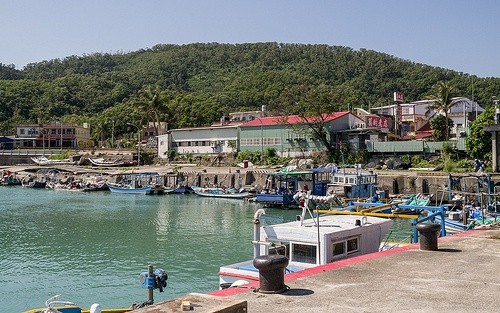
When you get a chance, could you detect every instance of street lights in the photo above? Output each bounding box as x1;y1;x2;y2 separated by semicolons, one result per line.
247;113;263;165
126;122;140;166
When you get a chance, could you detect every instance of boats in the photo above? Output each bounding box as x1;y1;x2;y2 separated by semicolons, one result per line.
0;168;257;198
217;214;408;291
254;152;500;231
